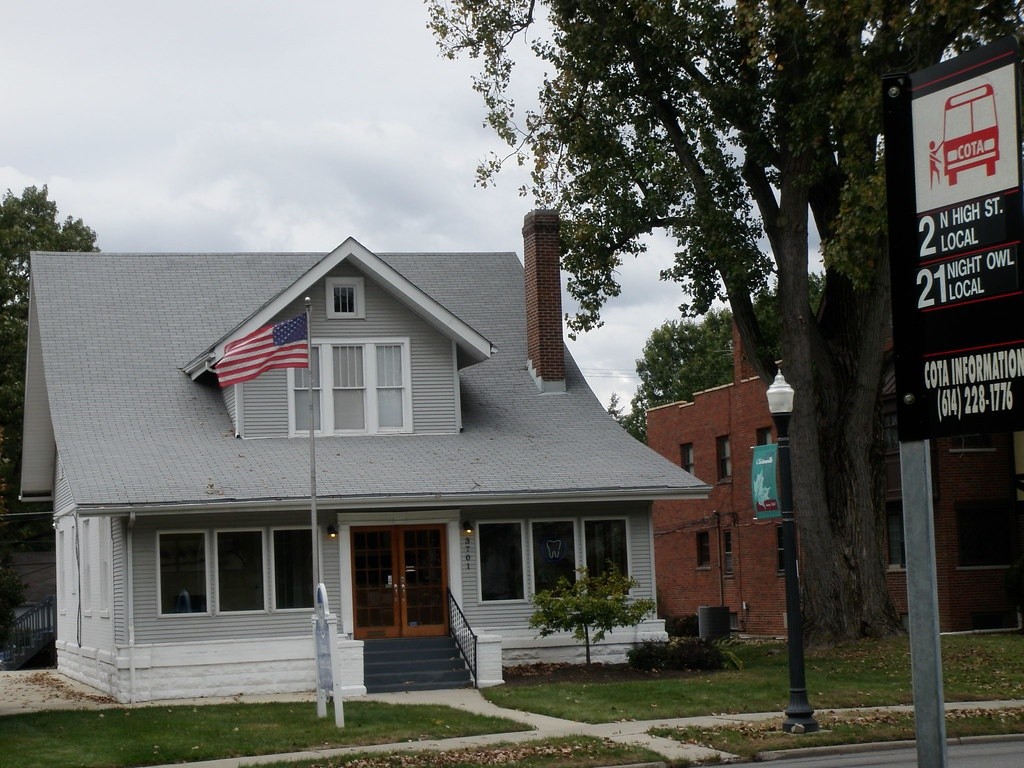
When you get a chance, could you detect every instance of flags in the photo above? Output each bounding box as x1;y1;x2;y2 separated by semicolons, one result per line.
215;313;309;387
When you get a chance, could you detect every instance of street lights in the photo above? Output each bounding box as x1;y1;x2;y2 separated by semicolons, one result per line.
765;369;820;735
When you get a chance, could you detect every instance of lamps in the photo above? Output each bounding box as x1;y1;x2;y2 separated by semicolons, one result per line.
463;520;475;534
328;525;338;538
